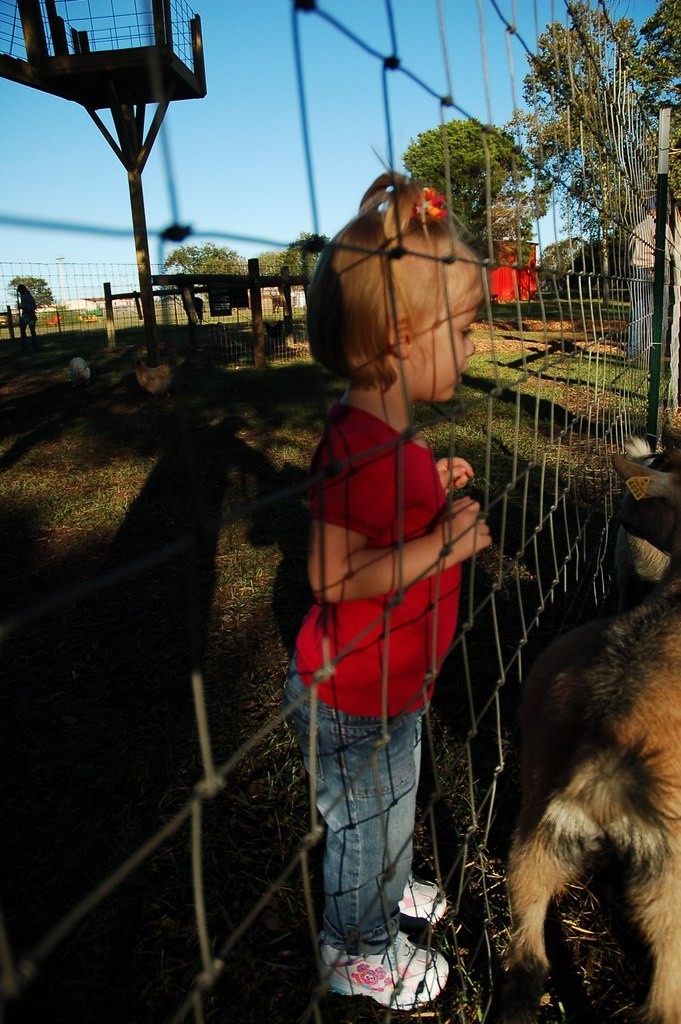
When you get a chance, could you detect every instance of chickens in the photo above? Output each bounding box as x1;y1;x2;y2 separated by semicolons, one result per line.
134;356;186;401
68;357;91;387
262;320;285;347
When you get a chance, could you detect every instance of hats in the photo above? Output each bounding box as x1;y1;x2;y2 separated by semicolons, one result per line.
646;196;670;210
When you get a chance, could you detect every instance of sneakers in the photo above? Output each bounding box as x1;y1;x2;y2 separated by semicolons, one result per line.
319;930;450;1011
397;870;447;926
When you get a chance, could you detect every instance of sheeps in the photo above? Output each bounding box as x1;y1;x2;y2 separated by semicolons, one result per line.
490;425;681;1024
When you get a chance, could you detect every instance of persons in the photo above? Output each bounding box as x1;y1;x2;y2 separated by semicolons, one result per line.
17;284;38;353
282;167;491;1011
624;199;674;362
